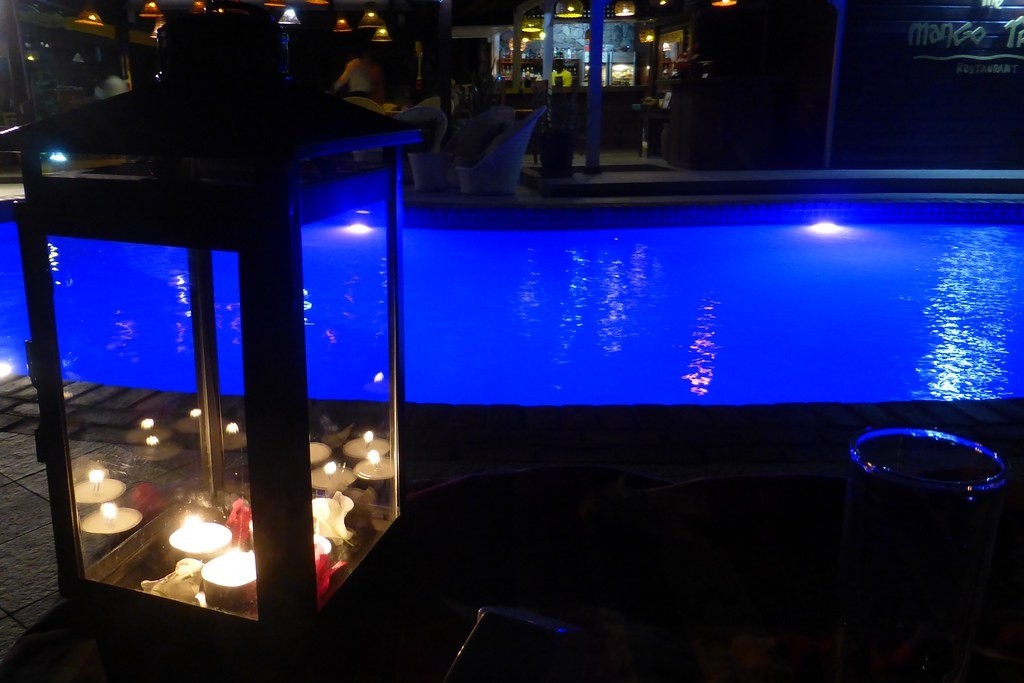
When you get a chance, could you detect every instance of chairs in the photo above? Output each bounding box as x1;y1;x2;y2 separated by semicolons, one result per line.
345;96;548;194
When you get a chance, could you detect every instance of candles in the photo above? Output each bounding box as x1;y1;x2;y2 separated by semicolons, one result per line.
72;468;142;561
169;515;257;615
310;429;394;497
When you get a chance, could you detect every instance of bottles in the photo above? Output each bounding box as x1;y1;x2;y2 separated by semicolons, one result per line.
500;45;580;87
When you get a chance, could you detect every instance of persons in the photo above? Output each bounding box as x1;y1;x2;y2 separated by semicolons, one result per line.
333;47;385;100
397;0;442;104
551;59;572;86
